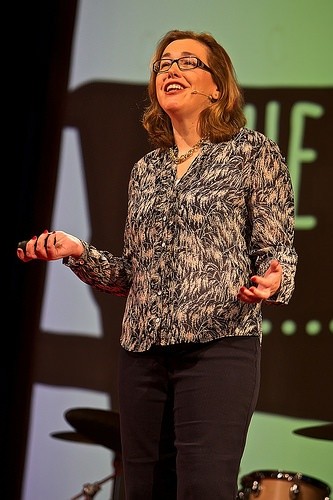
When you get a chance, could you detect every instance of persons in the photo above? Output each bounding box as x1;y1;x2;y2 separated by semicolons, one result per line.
18;29;297;500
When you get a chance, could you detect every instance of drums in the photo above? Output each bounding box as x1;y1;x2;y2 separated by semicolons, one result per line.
241;471;331;500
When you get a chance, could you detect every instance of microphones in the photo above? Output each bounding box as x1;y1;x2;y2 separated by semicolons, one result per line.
191;90;215;103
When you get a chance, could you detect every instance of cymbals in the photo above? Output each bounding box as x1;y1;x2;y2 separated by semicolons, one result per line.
50;407;118;452
293;423;333;442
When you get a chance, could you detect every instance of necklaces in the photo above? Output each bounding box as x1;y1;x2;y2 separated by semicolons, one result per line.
169;133;210;164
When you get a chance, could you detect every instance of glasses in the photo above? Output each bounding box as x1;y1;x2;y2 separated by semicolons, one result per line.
153;56;215;75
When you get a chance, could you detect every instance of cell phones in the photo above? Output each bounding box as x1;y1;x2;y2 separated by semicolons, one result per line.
17;233;56;256
